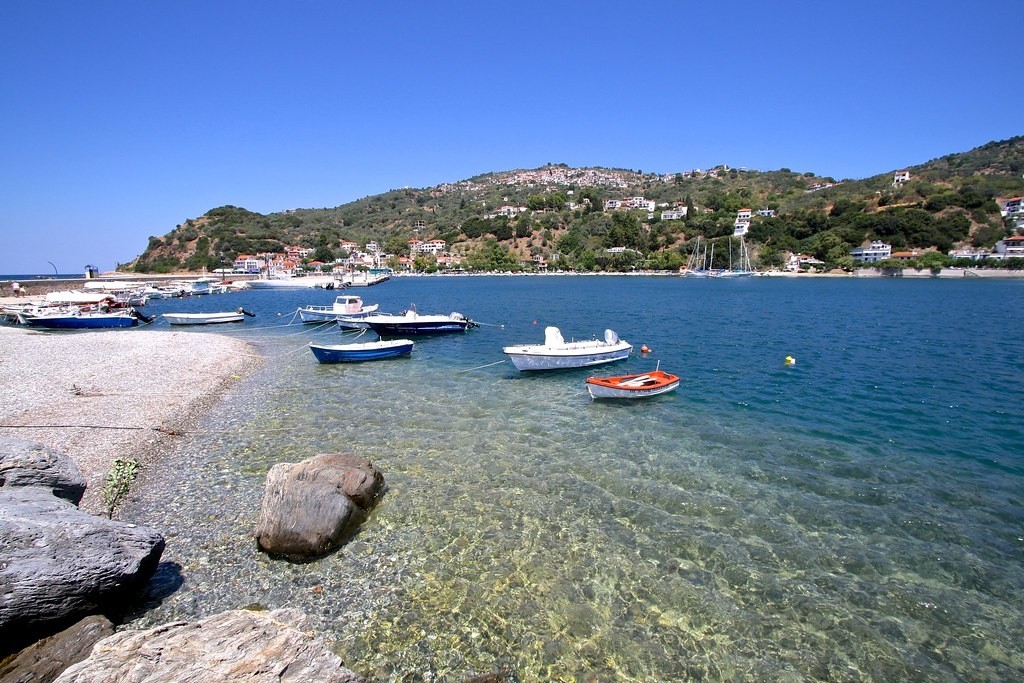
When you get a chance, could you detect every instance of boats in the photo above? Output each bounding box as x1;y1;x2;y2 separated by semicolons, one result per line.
0;280;252;327
297;294;380;322
333;312;394;330
308;334;415;364
161;307;256;325
364;303;481;336
584;359;681;401
502;326;634;372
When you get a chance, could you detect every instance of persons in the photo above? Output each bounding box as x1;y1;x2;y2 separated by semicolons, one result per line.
11;280;27;298
106;306;112;313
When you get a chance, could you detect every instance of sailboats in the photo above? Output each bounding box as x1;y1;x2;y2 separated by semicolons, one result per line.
680;235;768;278
